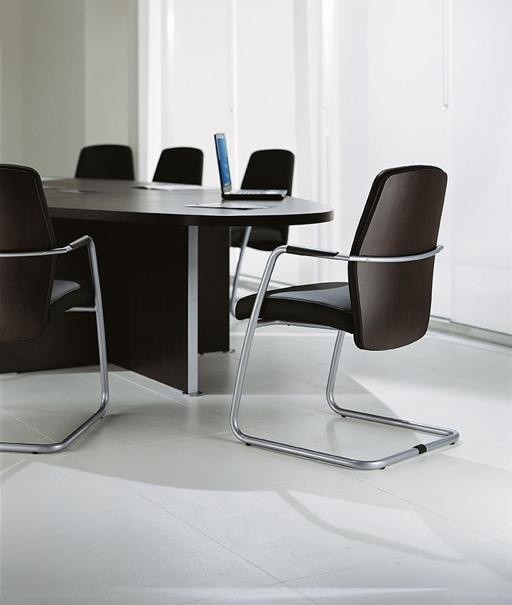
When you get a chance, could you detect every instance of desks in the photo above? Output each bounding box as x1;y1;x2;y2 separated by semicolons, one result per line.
43;175;334;397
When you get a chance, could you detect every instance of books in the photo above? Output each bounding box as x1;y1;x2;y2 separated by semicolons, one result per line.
131;182;205;191
183;201;270;209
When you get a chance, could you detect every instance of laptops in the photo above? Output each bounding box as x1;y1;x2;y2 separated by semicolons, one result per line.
214;133;288;200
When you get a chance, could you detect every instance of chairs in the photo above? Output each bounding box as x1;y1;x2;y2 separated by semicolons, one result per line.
149;146;206;188
220;146;295;332
72;142;137;182
0;159;115;451
227;166;460;469
